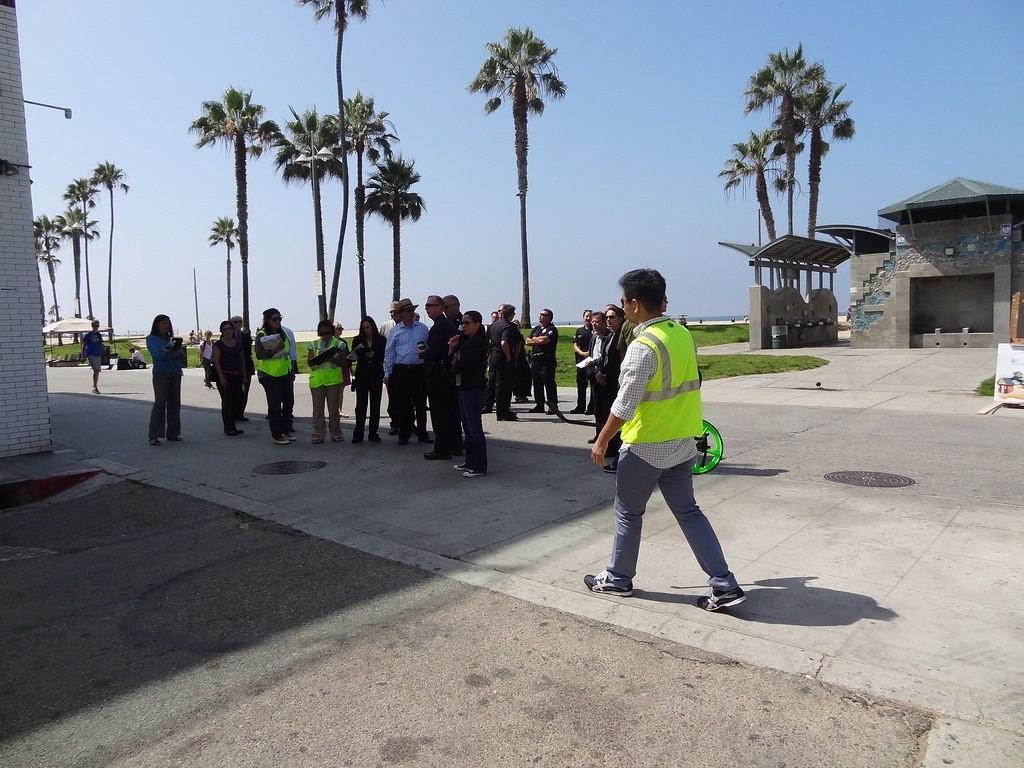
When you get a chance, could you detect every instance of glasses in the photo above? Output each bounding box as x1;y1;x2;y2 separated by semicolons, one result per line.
620;298;629;307
336;328;344;331
234;321;243;323
605;315;616;320
271;317;283;322
319;331;333;337
425;303;473;325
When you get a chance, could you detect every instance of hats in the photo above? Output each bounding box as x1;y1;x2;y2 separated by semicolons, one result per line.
395;298;419;311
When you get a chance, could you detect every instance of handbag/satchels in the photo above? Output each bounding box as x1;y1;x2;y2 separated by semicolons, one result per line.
207;361;218;382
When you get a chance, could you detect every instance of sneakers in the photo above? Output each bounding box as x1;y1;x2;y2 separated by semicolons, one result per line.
697;585;747;611
453;462;487;478
583;570;634;596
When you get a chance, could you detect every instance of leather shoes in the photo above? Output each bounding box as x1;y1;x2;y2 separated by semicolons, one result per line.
312;427;464;460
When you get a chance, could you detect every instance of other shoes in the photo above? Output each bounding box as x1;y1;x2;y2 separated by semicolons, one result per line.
481;406;493;414
529;404;545;413
272;434;297;444
209;386;215;389
93;388;100;394
148;436;161;446
236;416;249;422
497;411;518;421
602;457;618;473
227;430;244;436
166;436;182;441
546;404;558;415
570;405;594;415
587;434;598;443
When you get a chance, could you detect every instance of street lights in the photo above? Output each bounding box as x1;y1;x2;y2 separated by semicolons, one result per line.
63;223;85;347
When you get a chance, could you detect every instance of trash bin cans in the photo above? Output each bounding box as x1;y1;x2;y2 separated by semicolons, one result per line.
771;326;788;348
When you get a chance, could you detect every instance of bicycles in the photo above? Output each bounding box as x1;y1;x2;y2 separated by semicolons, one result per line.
692;420;725;475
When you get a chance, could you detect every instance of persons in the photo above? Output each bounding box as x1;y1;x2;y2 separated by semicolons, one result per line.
584;269;748;611
190;308;297;444
306;295;557;477
570;294;667;473
81;320;105;394
146;315;185;446
129;347;145;368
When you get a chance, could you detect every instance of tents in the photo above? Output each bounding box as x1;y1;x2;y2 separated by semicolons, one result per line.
43;316;117;357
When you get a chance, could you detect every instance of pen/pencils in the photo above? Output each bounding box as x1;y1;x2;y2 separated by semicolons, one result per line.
447;335;461;344
316;347;321;351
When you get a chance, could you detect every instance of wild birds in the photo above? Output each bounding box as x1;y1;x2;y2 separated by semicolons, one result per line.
816;382;821;388
